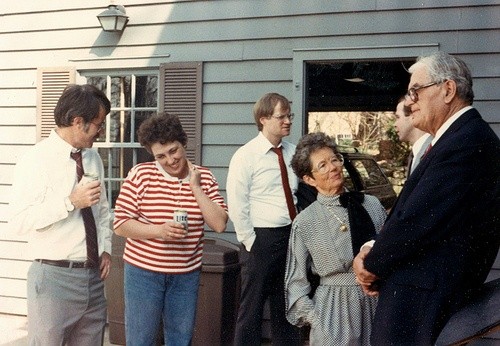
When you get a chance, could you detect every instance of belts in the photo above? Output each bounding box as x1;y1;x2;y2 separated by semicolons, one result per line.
34;259;99;268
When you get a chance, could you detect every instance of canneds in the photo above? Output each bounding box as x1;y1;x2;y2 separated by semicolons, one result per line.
173;210;188;231
82;173;98;186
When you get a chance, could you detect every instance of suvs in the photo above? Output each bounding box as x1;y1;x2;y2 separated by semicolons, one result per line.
338;151;397;214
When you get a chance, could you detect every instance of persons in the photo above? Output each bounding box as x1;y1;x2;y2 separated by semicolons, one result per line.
114;113;229;346
226;92;300;346
8;86;111;346
353;51;500;346
282;133;386;346
392;94;434;181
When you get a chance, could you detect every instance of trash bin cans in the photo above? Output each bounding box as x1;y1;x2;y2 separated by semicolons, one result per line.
106;219;240;346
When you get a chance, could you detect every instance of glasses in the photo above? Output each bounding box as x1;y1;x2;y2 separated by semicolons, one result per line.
310;153;344;175
272;113;295;120
89;120;105;132
407;79;447;103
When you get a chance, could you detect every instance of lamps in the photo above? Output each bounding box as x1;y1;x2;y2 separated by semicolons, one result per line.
96;5;129;32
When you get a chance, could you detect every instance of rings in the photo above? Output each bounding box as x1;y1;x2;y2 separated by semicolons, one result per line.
108;266;111;268
193;167;196;170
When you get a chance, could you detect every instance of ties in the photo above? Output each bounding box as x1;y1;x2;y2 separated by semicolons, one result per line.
420;143;432;162
70;149;98;266
407;153;414;178
270;146;297;222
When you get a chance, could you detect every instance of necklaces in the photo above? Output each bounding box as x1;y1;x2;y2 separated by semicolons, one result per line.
324;205;347;232
